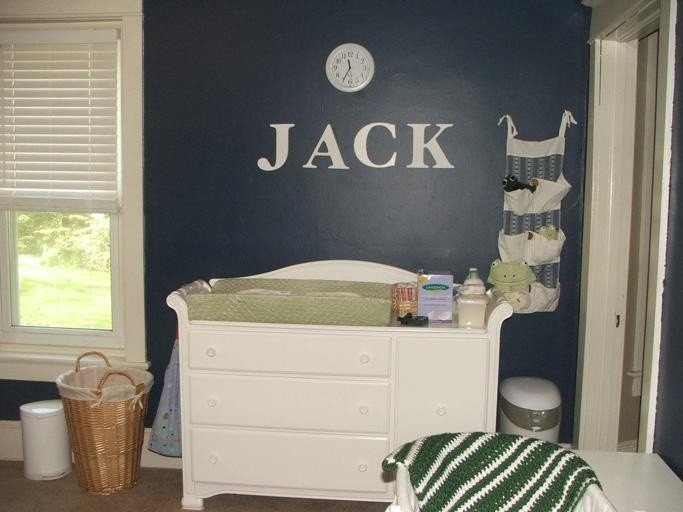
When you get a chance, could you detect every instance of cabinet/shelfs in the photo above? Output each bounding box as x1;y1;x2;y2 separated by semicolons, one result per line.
164;257;515;511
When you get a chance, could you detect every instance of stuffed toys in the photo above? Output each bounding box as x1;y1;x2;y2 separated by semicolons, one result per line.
501;173;535;195
486;258;537;310
534;222;559;242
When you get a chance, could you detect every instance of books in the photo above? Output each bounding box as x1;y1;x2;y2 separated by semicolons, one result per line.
416;270;453;325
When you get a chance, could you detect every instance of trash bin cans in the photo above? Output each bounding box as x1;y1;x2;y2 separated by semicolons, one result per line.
18;398;72;482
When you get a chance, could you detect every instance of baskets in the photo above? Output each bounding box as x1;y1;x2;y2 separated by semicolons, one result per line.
55;351;154;496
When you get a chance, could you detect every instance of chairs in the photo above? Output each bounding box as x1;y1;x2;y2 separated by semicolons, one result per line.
387;431;615;511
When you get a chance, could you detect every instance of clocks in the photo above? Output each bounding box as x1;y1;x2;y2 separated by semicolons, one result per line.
323;41;377;96
